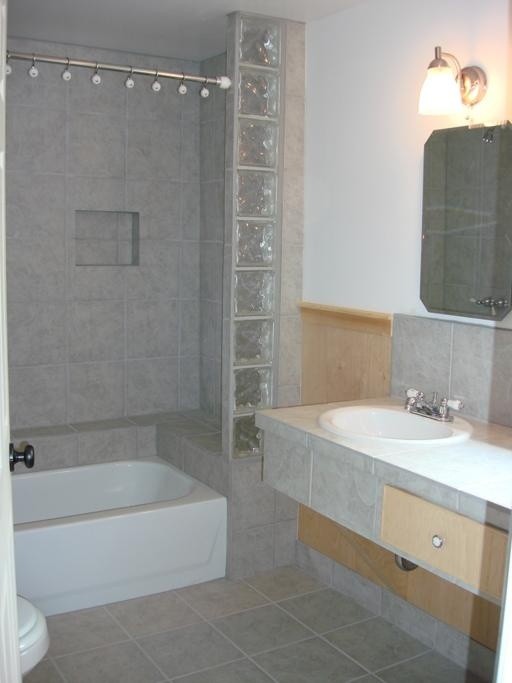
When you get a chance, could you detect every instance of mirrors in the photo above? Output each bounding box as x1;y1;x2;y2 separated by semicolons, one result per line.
420;122;511;321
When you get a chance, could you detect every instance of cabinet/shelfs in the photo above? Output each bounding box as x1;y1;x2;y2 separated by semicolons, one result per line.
379;484;508;604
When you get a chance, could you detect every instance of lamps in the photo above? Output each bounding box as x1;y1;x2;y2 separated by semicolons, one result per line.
418;47;488;117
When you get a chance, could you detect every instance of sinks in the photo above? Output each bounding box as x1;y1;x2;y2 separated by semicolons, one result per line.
315;401;476;446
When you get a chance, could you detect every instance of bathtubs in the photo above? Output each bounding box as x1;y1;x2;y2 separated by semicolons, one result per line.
8;454;228;613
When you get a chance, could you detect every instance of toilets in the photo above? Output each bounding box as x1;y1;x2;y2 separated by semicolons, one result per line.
16;595;50;679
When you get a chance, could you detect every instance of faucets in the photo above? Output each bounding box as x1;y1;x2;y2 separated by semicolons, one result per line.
404;393;439;416
